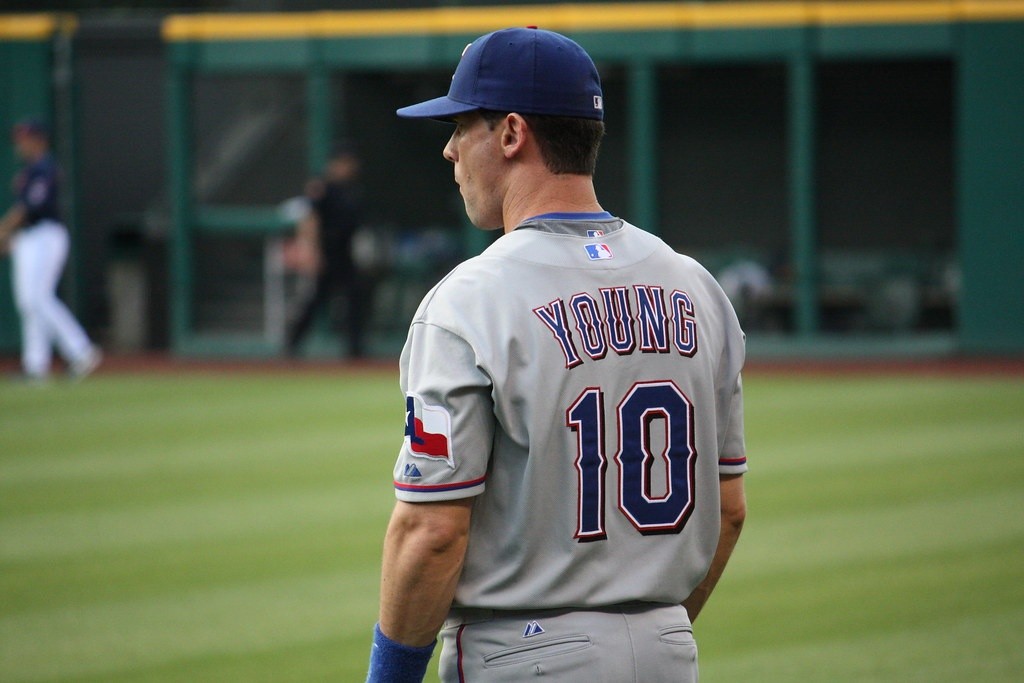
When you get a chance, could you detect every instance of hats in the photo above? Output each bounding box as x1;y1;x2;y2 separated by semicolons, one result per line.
396;25;604;120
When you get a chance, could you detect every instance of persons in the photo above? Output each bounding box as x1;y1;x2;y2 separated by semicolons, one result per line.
364;26;748;683
1;123;101;377
279;142;373;359
